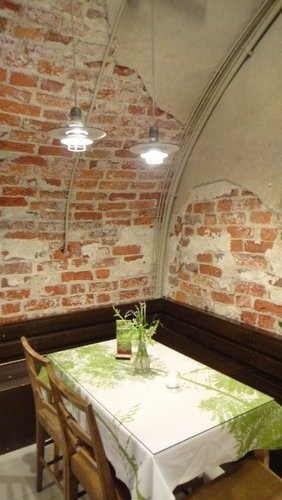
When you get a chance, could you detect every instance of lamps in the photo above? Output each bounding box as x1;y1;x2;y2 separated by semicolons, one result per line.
129;0;180;165
47;0;108;153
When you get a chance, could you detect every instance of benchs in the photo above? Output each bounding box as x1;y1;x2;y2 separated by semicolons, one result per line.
0;298;282;479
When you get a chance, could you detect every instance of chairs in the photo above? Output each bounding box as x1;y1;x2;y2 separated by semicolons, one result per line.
184;457;282;500
21;336;87;500
48;370;132;500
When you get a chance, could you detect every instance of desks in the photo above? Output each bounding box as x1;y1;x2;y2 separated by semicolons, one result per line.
39;337;282;500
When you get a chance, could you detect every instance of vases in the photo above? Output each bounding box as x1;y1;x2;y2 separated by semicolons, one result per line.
133;342;150;372
